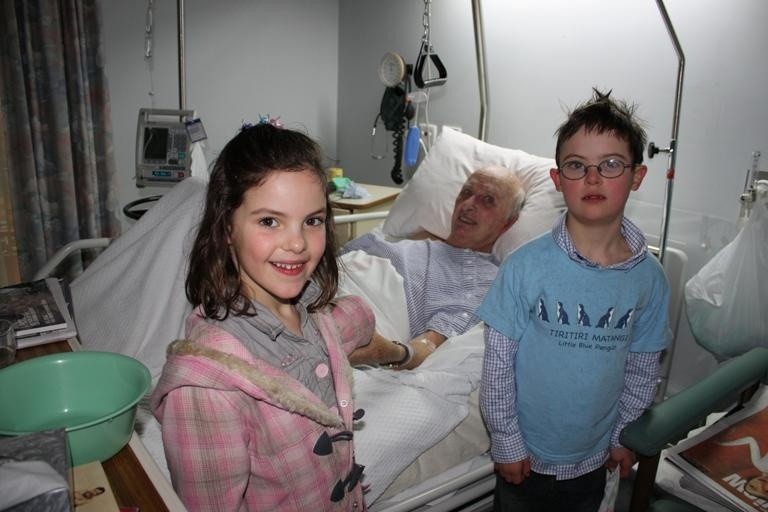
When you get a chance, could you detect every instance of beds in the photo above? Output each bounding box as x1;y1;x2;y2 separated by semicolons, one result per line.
35;211;696;511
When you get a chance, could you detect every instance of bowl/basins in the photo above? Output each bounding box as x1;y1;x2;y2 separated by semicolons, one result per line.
3;354;150;466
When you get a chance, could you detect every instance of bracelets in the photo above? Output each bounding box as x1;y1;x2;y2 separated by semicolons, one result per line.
379;337;415;369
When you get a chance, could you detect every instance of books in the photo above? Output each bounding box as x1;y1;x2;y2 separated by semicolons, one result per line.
665;395;767;512
1;277;66;336
9;278;78;349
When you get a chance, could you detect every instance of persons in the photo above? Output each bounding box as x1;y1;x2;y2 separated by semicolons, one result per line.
745;474;768;502
334;162;529;375
164;117;379;511
475;88;670;511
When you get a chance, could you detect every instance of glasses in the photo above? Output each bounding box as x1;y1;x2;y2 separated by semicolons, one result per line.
558;159;635;180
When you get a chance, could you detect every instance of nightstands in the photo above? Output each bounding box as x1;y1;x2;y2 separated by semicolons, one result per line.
326;180;402;252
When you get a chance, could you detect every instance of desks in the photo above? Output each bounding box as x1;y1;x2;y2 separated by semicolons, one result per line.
0;274;169;512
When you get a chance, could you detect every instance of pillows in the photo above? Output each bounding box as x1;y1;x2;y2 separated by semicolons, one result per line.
386;119;573;262
